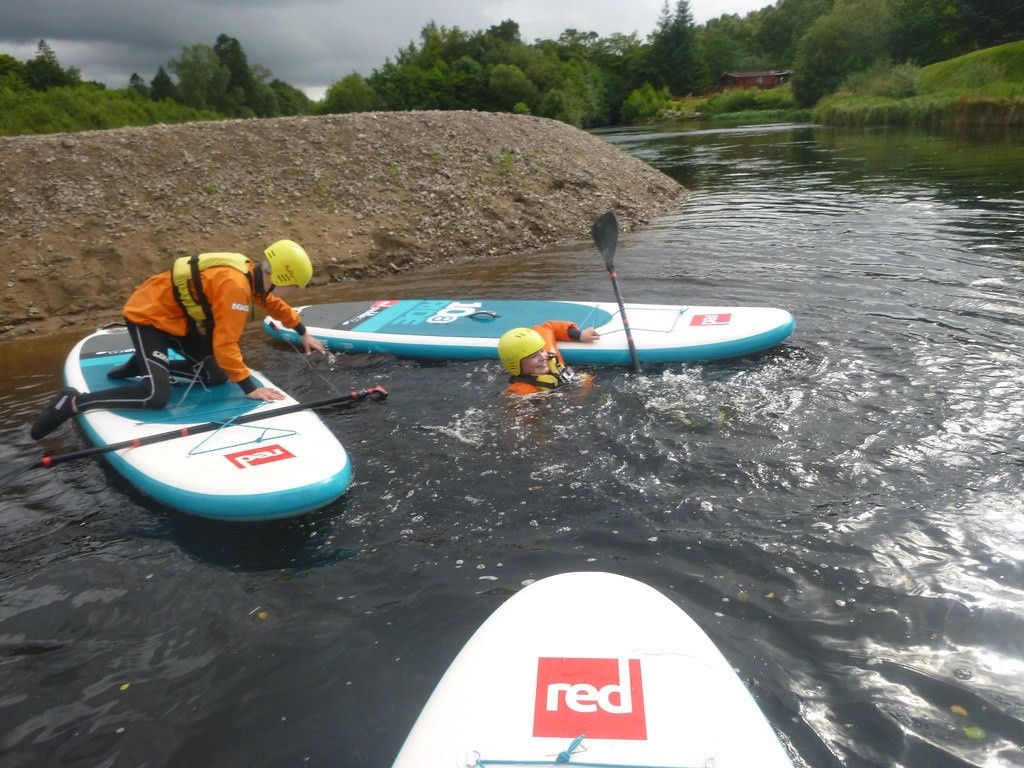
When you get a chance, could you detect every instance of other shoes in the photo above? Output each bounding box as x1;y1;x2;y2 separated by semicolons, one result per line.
107;353;140;381
30;386;80;441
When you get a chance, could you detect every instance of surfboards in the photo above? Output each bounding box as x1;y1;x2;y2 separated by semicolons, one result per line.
389;571;798;767
263;299;797;366
62;324;353;520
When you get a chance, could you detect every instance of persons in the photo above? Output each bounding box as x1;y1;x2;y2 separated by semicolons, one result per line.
497;320;601;399
31;239;327;440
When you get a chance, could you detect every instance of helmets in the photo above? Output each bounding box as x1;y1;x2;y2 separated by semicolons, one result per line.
263;240;312;289
497;328;546;375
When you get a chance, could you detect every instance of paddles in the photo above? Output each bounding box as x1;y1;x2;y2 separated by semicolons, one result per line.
586;212;647;378
0;382;390;501
